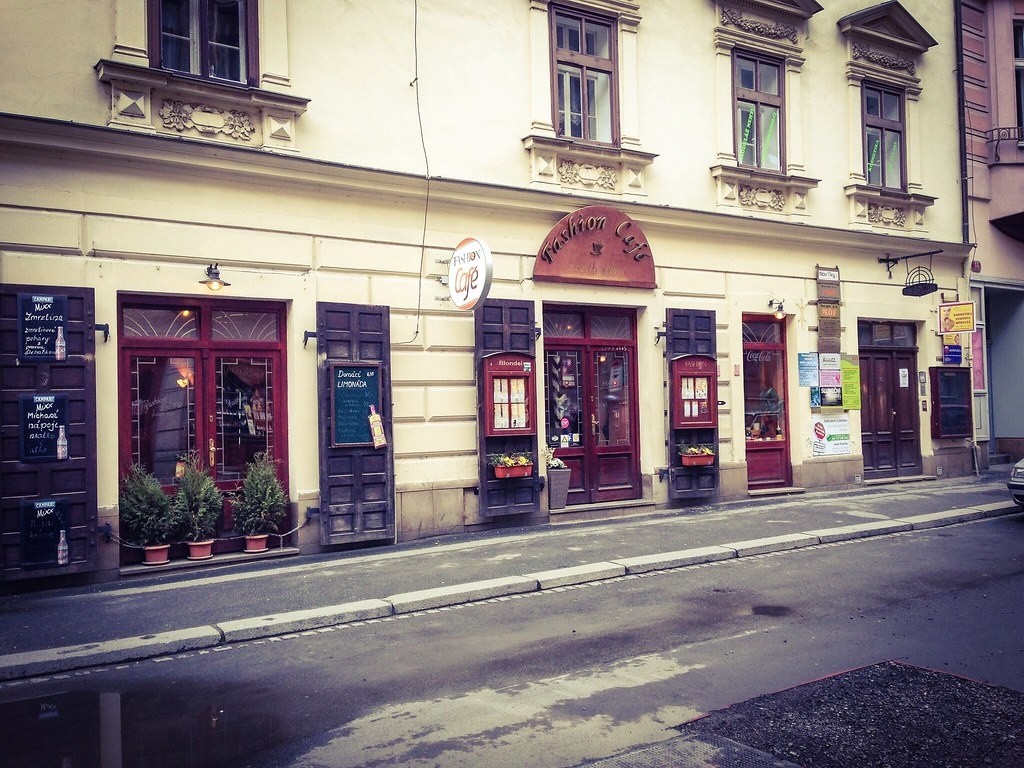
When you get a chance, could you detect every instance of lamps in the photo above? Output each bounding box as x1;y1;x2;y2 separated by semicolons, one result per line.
199;262;231;290
768;298;786;319
176;371;192;388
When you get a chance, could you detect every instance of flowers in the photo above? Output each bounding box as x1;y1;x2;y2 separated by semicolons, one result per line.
678;441;714;455
488;449;532;467
541;446;568;469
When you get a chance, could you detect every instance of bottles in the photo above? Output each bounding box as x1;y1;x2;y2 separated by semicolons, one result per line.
54;326;66;361
57;530;68;565
776;419;782;440
56;425;68;460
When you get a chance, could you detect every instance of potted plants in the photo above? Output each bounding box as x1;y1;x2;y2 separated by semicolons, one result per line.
118;460;173;563
173;446;223;559
227;447;290;553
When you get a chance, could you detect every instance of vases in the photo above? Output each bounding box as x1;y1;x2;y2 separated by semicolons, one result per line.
547;469;572;510
683;454;714;466
496;464;533;478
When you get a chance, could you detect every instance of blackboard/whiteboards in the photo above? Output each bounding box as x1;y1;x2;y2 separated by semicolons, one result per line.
19;393;69;462
15;292;68;362
18;497;71;570
330;362;388;450
